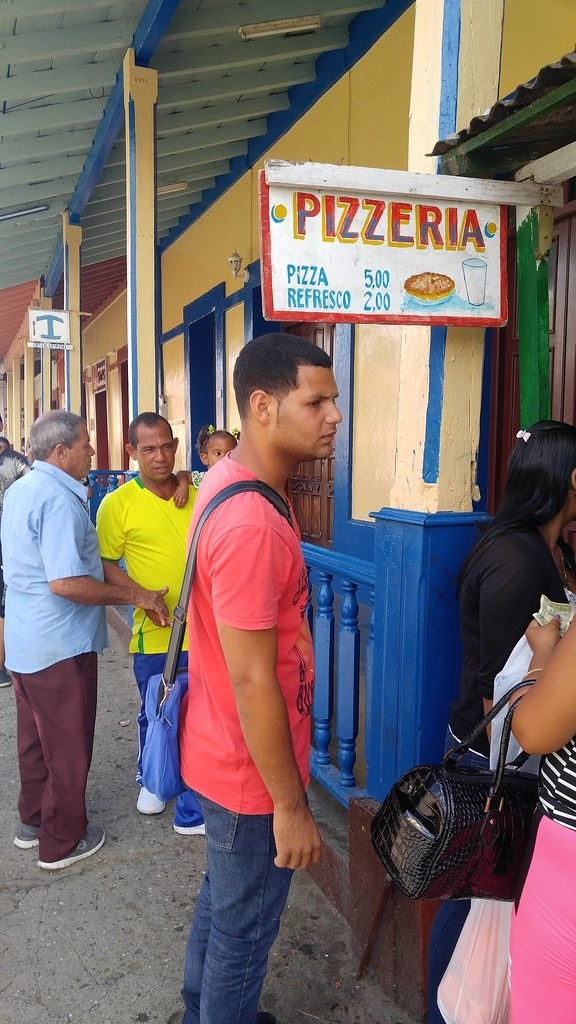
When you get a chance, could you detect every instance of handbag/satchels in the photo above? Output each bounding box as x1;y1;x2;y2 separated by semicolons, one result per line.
437;899;513;1023
371;678;541;902
142;666;189;804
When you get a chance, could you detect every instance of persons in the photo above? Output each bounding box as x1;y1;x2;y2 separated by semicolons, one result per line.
425;419;576;1024
0;436;10;449
175;332;342;1023
96;412;211;835
1;417;32;687
506;594;575;1023
0;409;173;870
173;425;242;509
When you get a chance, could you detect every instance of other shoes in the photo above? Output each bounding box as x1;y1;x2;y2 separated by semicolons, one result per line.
257;1012;277;1024
0;668;13;687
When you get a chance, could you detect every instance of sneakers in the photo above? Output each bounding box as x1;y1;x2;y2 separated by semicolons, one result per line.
136;786;167;815
173;824;205;836
13;825;39;849
37;826;105;870
